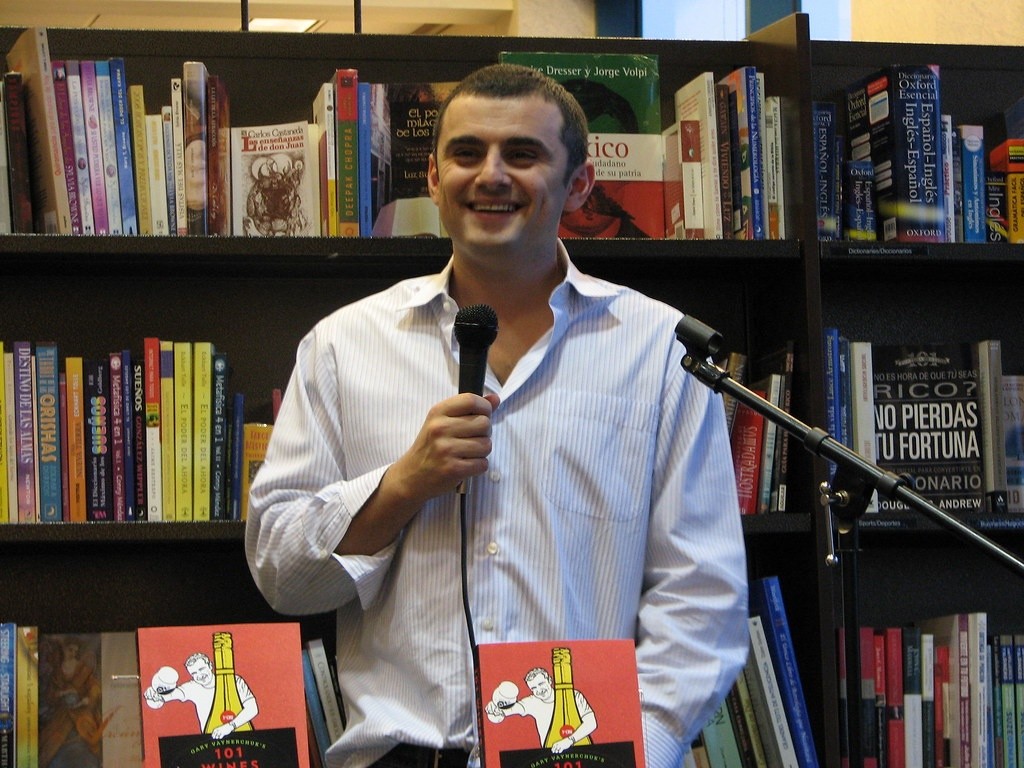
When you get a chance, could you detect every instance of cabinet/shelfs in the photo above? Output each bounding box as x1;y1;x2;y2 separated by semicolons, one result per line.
0;13;1024;768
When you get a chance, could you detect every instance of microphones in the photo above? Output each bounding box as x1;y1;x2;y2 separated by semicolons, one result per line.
453;304;500;494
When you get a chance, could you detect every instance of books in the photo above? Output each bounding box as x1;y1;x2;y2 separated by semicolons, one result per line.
717;329;1024;515
499;52;1024;241
477;639;644;768
0;24;463;237
0;623;348;768
0;339;281;524
691;577;1024;768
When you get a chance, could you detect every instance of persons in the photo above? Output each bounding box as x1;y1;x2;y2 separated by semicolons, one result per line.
245;64;750;768
